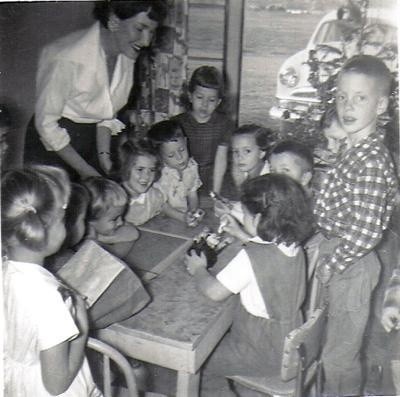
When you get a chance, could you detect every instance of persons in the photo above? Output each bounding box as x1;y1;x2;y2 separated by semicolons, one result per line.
170;65;232;198
183;103;400;397
1;163;150;396
23;1;167;185
117;127;205;228
306;52;400;396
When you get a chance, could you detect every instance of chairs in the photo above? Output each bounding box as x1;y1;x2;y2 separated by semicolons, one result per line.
69;335;138;396
222;303;331;397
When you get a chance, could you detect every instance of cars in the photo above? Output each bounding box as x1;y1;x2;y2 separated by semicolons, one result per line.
267;6;396;138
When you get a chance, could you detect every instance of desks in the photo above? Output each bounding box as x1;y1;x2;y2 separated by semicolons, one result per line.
58;170;241;396
